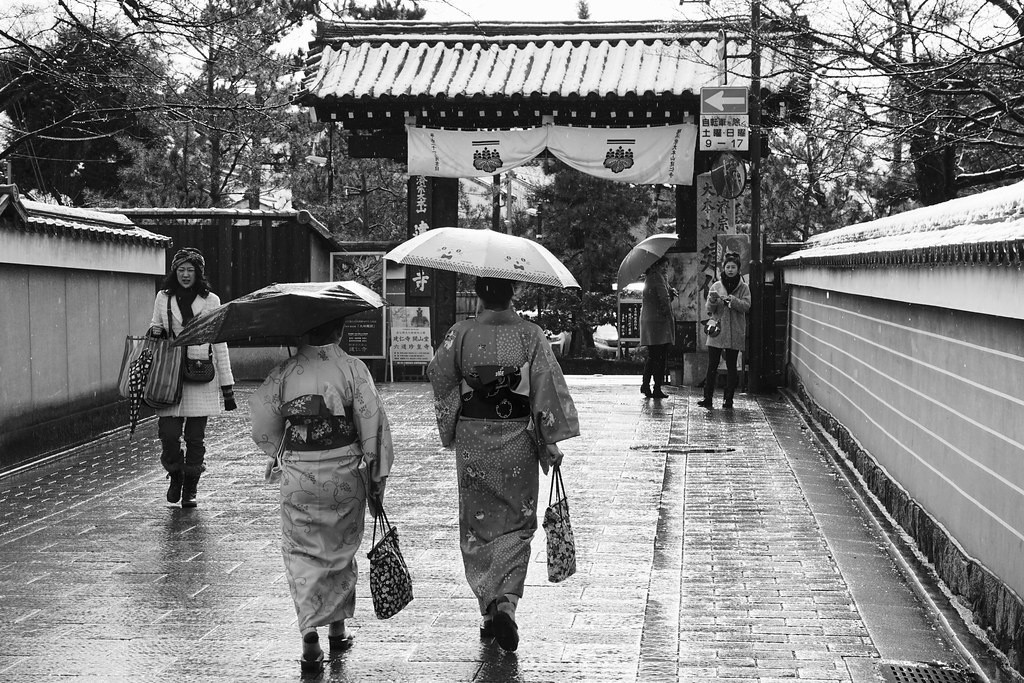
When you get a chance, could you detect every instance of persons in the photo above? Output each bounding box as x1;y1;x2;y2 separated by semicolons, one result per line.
149;247;237;507
696;251;751;408
248;321;394;672
427;274;581;652
641;256;677;398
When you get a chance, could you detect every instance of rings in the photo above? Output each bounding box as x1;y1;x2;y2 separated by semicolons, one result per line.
154;332;156;335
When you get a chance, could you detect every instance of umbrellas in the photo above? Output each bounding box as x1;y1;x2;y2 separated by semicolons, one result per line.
617;233;681;300
170;280;393;358
129;327;154;442
382;227;580;288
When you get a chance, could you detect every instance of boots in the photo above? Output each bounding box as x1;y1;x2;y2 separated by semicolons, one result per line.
160;448;184;502
182;465;206;507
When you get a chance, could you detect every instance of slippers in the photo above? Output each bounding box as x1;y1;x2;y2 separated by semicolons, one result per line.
492;612;520;651
327;628;356;649
300;648;324;676
480;618;494;638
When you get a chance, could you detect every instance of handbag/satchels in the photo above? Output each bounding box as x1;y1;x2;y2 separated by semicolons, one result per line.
118;326;183;408
704;320;721;337
543;467;577;583
180;357;215;382
366;496;414;618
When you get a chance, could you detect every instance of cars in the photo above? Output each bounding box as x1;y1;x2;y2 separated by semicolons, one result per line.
516;309;572;360
591;311;644;363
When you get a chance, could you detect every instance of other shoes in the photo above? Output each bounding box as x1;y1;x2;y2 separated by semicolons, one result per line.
723;398;734;407
653;388;669;398
698;397;713;407
641;385;653;398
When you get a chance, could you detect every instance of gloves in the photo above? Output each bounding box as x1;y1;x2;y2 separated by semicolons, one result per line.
220;383;237;411
